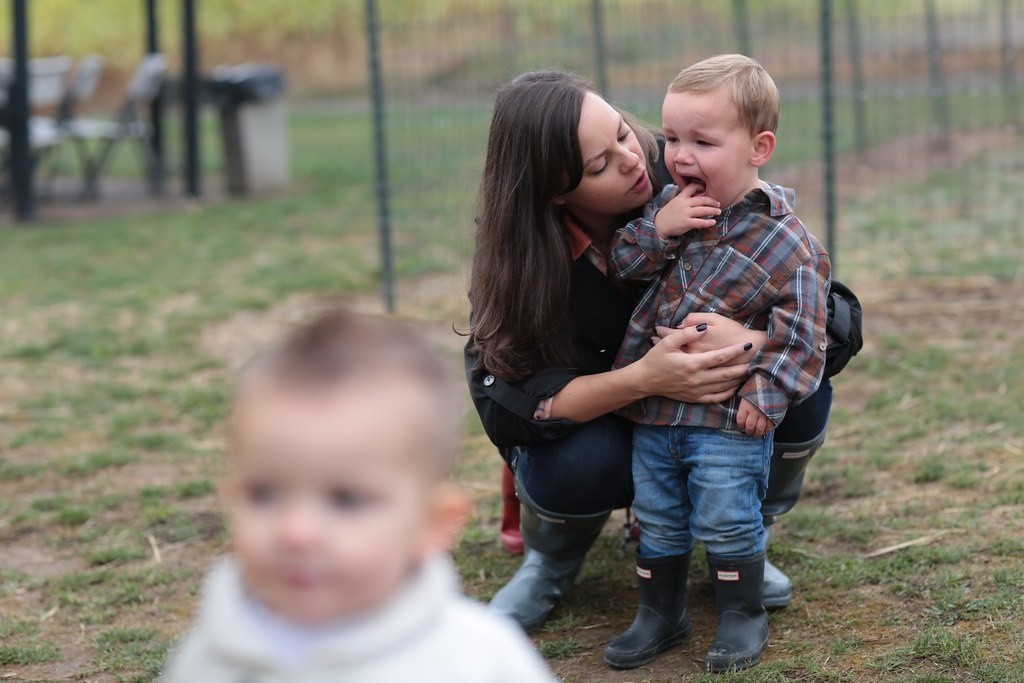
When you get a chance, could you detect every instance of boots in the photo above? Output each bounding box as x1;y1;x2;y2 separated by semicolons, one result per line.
489;462;612;639
601;544;693;668
759;427;830;608
705;552;770;670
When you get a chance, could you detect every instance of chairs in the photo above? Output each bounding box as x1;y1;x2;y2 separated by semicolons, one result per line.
0;53;171;204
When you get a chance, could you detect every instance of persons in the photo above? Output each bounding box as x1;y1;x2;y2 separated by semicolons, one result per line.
602;53;833;675
164;311;559;683
450;69;865;630
